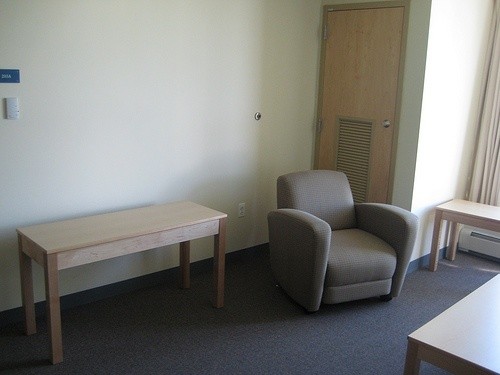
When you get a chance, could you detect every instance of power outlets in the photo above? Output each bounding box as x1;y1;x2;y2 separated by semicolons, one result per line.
239;203;246;216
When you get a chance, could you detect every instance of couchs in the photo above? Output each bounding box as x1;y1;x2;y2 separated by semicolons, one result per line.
267;169;420;316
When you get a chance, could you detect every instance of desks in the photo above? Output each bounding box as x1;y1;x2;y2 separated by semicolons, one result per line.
427;197;500;273
402;273;499;375
18;199;230;364
